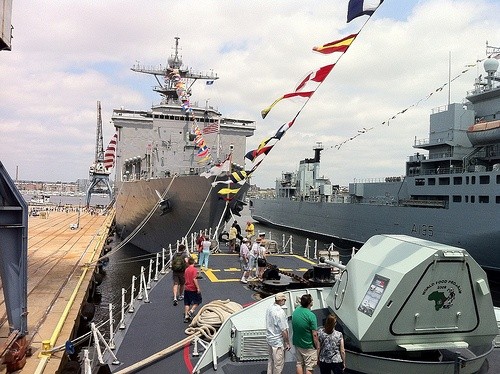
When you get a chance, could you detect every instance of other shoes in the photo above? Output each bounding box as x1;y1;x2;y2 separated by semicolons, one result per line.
241;278;248;283
178;296;184;301
187;310;196;318
173;300;178;306
184;317;190;323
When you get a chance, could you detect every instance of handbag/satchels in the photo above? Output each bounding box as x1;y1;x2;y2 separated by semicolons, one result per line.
203;250;209;254
258;258;266;267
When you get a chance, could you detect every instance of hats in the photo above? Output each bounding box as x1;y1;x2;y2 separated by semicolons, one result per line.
243;238;248;242
256;238;262;243
261;240;267;245
275;293;287;300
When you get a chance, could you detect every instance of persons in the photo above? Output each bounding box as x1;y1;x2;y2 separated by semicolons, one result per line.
317;315;346;374
171;245;190;307
195;233;212;271
292;294;320;374
266;293;291;374
230;220;269;283
183;258;202;324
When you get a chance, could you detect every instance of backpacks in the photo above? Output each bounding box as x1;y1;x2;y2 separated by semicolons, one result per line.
172;253;185;271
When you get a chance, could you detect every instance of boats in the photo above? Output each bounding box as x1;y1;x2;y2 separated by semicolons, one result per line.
77;37;500;374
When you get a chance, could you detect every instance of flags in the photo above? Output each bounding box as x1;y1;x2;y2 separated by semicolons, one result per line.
312;34;357;54
104;133;116;170
294;64;336;92
347;0;385;24
217;187;240;195
226;170;252;183
200;159;231;179
259;120;293;151
202;121;219;135
261;91;315;119
244;145;274;161
176;79;211;163
